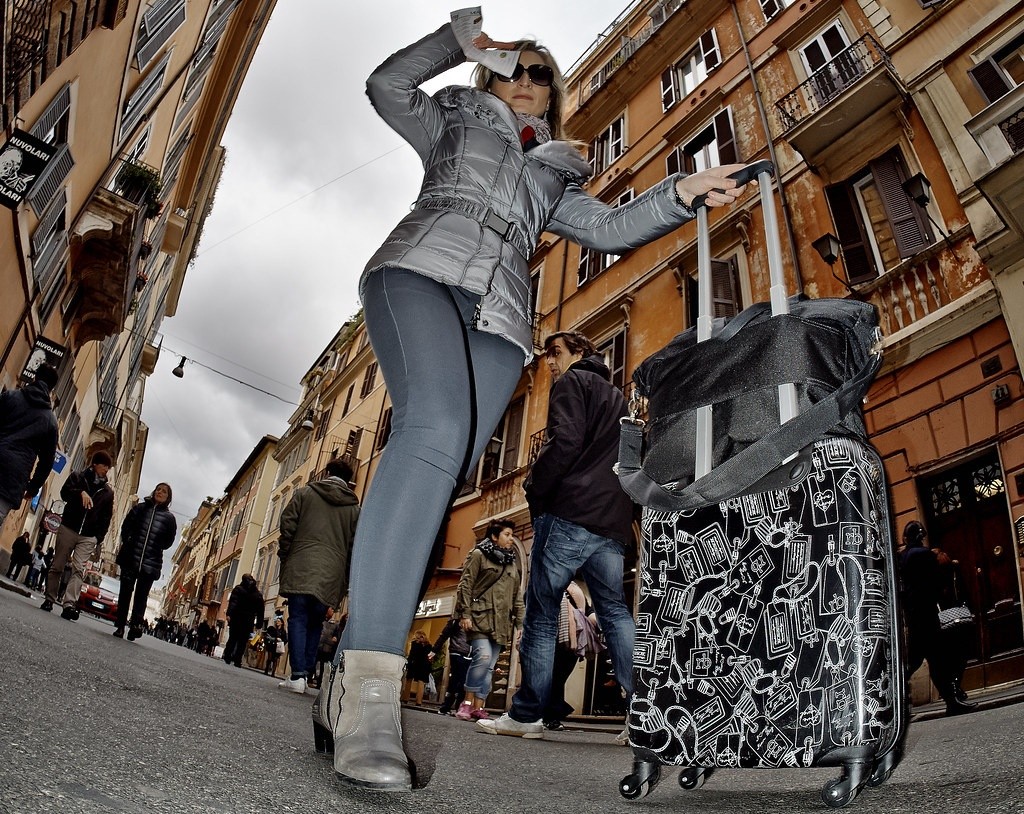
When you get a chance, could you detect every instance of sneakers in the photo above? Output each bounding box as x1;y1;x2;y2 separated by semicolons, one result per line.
613;729;630;747
475;712;544;739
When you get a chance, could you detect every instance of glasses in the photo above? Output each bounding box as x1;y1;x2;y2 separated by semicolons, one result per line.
497;63;554;86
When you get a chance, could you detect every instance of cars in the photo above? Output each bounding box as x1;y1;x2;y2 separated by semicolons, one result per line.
74;570;121;627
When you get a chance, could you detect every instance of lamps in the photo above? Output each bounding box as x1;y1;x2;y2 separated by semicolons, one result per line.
810;232;863;303
302;410;315;431
172;356;188;378
901;172;960;261
485;433;522;478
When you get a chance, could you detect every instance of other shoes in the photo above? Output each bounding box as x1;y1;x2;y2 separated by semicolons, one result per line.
127;627;142;641
144;631;212;658
224;658;275;677
5;574;42;591
946;700;978;716
956;689;968;702
41;600;53;611
440;702;449;713
416;703;421;706
113;628;124;639
472;708;496;719
400;699;408;704
456;701;473;720
61;607;80;620
546;721;565;731
278;676;305;694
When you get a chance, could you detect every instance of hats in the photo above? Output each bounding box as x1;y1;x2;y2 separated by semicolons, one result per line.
92;451;112;467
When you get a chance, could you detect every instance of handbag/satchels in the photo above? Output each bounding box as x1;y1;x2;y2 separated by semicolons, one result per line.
455;602;463;613
276;641;285;653
937;605;974;630
216;641;219;646
613;291;884;511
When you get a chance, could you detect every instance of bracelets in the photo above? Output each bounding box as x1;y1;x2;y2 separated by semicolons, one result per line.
675;183;692;211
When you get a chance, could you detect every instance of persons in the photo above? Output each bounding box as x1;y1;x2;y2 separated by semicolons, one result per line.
6;532;54;591
896;520;979;716
542;580;597;730
0;361;59;527
310;22;759;792
310;607;348;690
143;616;219;657
453;519;525;720
403;630;433;706
57;557;73;604
276;460;362;694
428;618;472;715
475;330;635;738
264;617;288;676
114;483;177;642
225;574;264;668
42;451;114;621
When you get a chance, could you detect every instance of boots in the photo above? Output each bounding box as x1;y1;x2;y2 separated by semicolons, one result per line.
313;649;413;793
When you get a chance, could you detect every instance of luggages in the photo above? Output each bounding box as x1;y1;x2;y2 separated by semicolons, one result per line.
615;159;910;809
